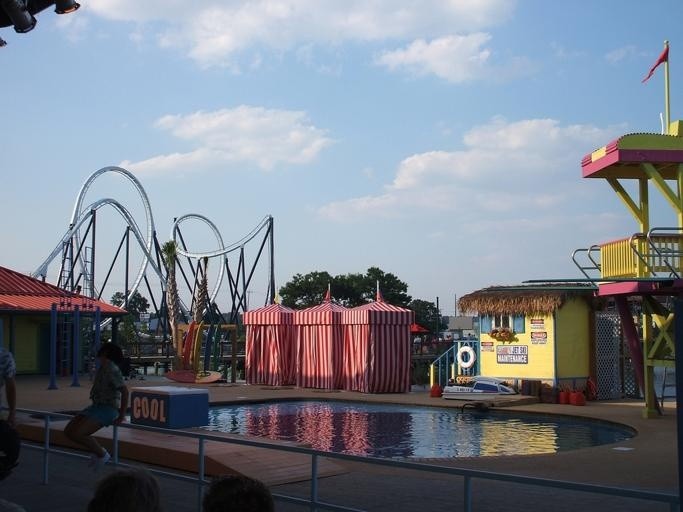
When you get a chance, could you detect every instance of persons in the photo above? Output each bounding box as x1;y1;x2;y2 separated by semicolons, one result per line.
62;342;129;466
200;472;276;511
0;344;16;479
86;465;160;511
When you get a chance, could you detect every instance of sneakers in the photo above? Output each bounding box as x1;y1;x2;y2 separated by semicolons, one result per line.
88;450;111;470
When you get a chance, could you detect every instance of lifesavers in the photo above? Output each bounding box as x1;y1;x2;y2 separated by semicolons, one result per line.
457;346;476;368
